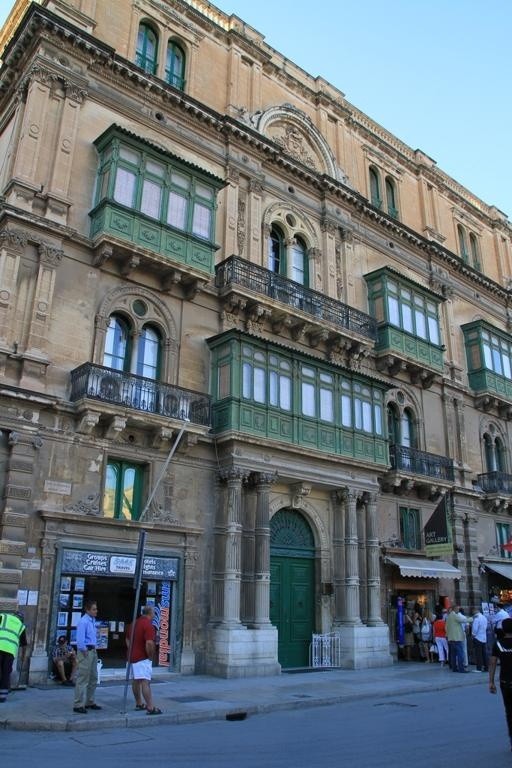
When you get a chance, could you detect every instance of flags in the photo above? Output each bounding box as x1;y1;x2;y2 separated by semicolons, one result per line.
424;495;454;556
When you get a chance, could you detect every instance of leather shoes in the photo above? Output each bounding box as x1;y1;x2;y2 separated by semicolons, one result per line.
85;703;101;710
73;707;87;712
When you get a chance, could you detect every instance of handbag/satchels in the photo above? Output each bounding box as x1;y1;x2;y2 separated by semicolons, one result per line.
429;645;439;654
405;623;429;634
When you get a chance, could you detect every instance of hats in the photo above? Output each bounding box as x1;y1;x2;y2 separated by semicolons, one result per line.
58;635;67;645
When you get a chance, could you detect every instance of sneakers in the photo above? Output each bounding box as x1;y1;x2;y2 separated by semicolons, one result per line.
440;661;489;673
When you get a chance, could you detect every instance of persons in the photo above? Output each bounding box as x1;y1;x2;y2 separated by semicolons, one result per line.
404;603;510;672
51;636;77;685
489;618;512;742
0;611;28;702
126;608;162;714
73;601;102;713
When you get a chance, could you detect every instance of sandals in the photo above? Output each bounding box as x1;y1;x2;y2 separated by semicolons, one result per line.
146;706;163;714
135;704;146;711
